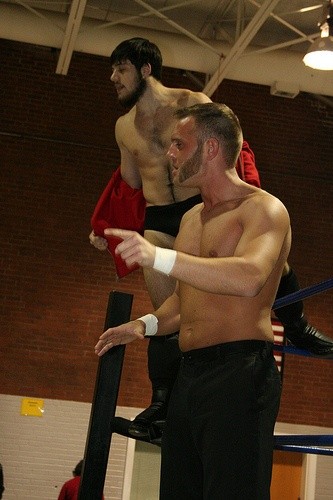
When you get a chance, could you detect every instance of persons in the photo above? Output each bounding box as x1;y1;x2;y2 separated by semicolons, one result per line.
87;36;332;436
57;459;104;500
90;102;292;500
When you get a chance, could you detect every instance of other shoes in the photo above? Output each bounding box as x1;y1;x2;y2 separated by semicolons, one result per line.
282;313;333;355
129;389;167;438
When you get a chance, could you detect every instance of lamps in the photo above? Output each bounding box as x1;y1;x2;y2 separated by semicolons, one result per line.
302;22;333;71
